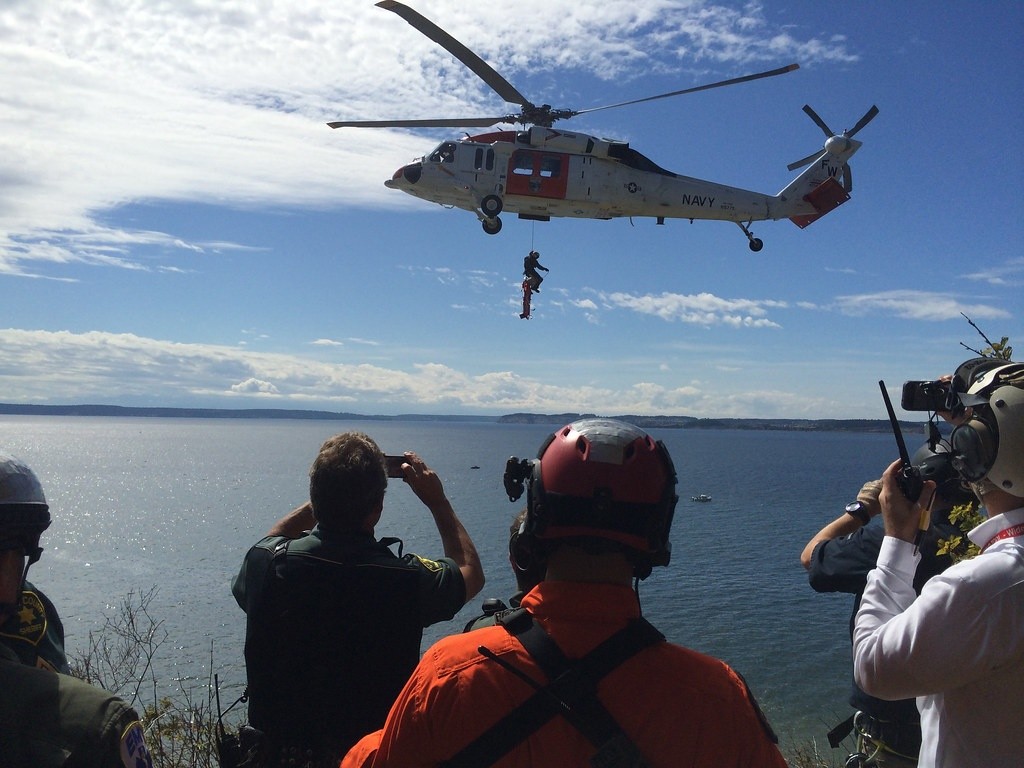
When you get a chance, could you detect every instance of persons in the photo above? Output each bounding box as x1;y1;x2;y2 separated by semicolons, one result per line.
440;144;456;163
232;431;485;768
0;452;156;768
799;357;1024;768
524;251;549;294
342;419;787;768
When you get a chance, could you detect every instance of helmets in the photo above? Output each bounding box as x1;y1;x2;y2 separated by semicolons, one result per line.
950;358;1016;419
532;252;539;259
947;362;1024;497
0;461;52;549
503;418;679;566
910;436;972;506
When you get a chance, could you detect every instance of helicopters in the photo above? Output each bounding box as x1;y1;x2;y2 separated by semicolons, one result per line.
325;0;879;252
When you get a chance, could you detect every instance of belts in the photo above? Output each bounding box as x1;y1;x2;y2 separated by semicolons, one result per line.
857;714;921;746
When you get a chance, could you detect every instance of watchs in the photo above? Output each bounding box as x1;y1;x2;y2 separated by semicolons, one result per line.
846;501;871;525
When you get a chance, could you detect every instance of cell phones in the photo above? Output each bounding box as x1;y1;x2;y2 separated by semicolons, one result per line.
385;456;411;478
901;381;951;411
